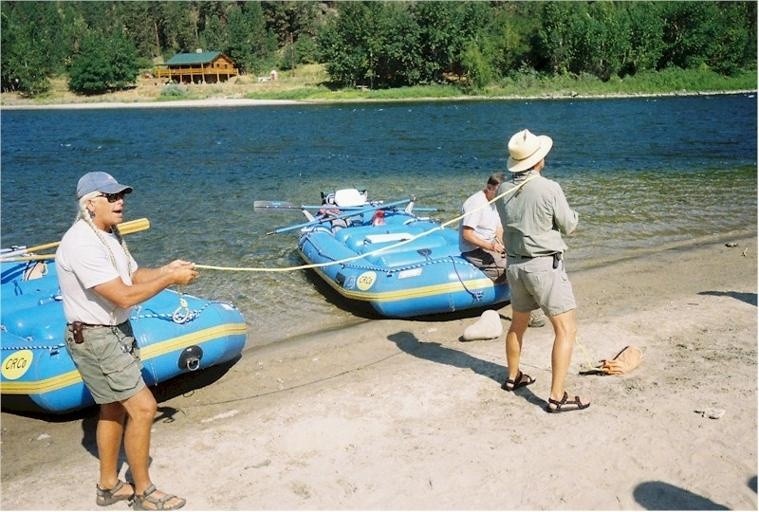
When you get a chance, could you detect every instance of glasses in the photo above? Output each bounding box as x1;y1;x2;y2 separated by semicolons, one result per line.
96;191;125;204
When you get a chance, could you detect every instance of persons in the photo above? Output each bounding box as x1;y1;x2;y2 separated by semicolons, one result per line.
55;171;198;510
459;173;544;327
494;129;590;413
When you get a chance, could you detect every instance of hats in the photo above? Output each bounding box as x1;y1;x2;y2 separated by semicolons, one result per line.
76;171;133;200
506;128;553;174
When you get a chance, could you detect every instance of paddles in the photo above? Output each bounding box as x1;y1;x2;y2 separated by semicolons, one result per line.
265;187;445;236
254;199;444;217
1;217;150;258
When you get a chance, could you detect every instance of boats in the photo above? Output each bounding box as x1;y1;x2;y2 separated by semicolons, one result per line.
297;203;510;319
1;247;247;416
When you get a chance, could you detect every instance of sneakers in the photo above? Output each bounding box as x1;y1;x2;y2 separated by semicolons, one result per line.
528;309;546;327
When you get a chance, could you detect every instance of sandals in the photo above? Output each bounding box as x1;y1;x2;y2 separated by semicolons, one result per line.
504;371;536;391
97;479;136;506
546;391;591;414
133;484;187;511
